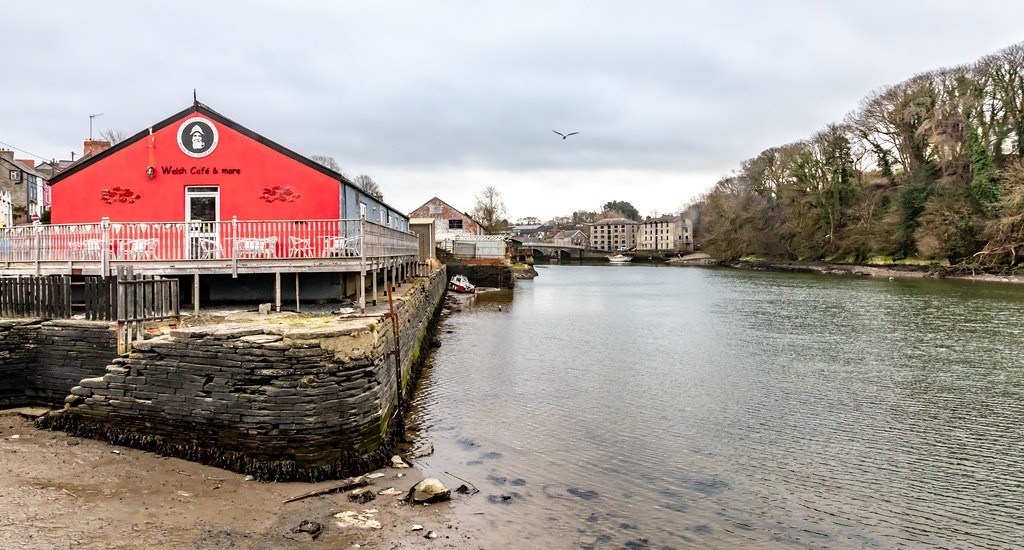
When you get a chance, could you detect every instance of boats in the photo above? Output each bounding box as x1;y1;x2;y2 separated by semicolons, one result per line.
449;276;476;293
606;253;634;262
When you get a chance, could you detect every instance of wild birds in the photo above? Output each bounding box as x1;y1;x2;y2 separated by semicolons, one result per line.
551;128;580;140
